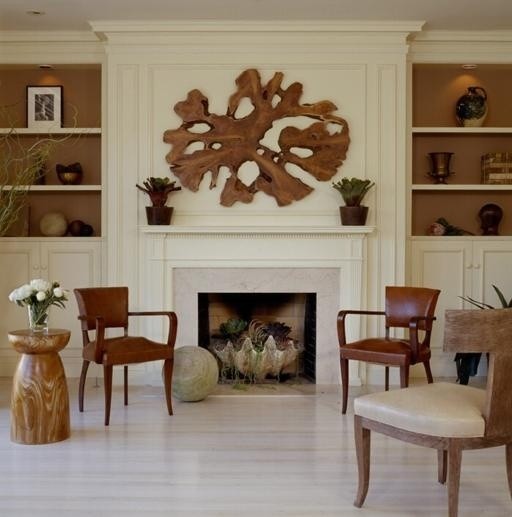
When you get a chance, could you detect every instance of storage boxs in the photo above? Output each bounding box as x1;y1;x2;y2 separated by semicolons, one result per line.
481;152;512;184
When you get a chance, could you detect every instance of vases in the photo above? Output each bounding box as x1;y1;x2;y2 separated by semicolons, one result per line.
27;304;49;332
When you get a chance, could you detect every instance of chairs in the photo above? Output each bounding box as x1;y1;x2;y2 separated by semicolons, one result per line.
73;287;177;425
353;308;512;517
337;287;440;414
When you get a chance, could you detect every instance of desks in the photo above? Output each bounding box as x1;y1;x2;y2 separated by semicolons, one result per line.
7;329;70;445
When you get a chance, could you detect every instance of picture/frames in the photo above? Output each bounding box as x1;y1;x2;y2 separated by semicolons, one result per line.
26;85;63;128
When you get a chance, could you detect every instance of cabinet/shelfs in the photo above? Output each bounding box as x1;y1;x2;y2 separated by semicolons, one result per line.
406;54;512;378
0;52;108;378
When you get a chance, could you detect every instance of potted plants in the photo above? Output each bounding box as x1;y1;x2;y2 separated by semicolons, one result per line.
136;177;181;225
332;178;374;226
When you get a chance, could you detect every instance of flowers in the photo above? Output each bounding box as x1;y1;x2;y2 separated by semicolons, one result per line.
8;279;70;308
